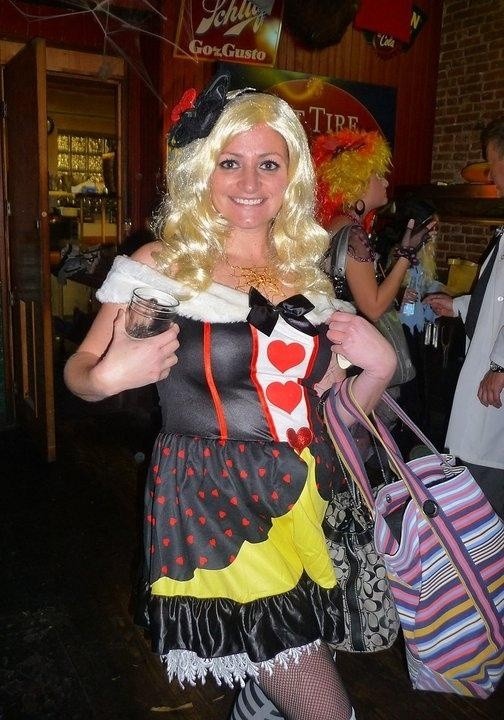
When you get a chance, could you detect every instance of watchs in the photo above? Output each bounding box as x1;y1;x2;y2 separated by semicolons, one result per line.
488;362;504;374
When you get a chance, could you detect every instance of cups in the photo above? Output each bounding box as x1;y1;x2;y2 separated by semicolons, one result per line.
125;286;183;339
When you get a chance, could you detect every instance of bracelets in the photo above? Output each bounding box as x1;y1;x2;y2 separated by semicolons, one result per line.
395;247;419;266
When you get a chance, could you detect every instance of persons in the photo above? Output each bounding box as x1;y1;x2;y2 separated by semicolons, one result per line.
61;72;399;719
100;135;117;197
419;118;503;521
303;129;438;439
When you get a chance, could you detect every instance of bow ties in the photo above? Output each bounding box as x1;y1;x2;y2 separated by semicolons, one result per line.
244;285;322;338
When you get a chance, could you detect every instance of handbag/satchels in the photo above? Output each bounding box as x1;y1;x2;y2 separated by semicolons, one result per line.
373;308;417;389
370;453;504;700
